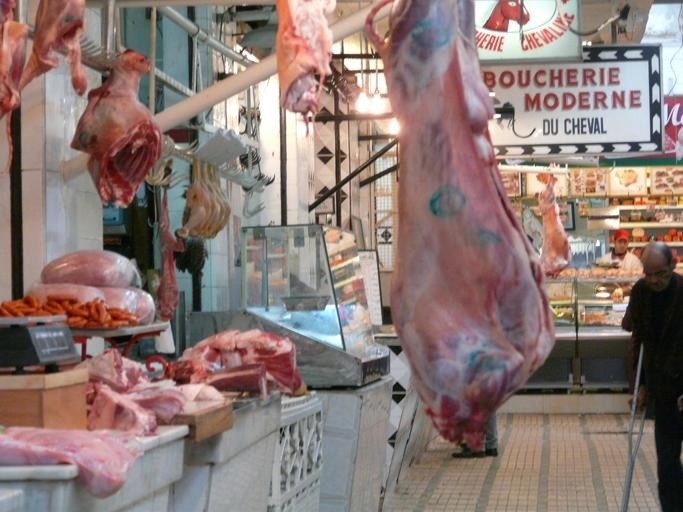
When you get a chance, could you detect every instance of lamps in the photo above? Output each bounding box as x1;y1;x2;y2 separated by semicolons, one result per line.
355;1;371;112
372;59;384;113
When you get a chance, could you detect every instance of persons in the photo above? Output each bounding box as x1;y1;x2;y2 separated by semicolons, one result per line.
621;241;682;512
591;228;644;314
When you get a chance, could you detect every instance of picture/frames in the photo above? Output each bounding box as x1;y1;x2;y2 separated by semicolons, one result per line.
564;201;575;232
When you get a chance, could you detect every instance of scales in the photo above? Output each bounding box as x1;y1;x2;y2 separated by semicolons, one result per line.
0;315;81;376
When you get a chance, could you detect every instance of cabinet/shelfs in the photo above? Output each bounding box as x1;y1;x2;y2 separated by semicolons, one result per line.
609;204;683;248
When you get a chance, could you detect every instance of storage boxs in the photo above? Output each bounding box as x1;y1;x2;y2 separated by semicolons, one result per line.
1;370;88;433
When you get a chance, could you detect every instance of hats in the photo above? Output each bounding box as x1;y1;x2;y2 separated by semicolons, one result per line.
615;230;630;241
